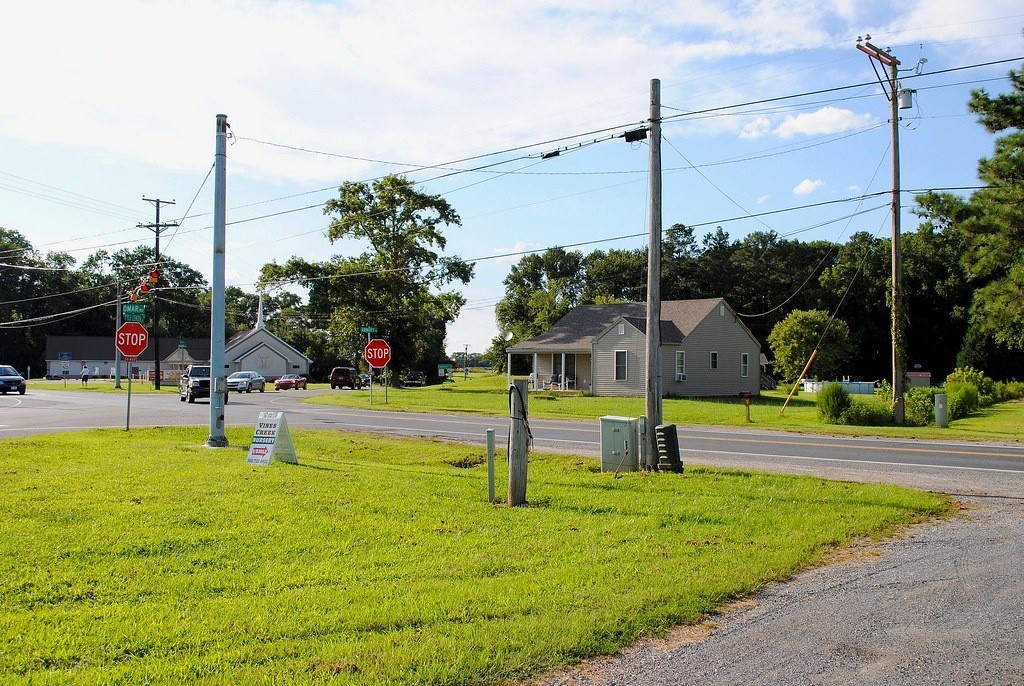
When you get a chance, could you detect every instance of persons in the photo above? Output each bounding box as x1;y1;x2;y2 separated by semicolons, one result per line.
528;370;539;382
81;365;90;387
550;372;562;383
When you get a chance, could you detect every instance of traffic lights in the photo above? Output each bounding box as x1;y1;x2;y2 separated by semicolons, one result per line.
128;270;159;300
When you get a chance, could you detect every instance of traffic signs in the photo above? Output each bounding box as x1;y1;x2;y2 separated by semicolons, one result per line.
178;341;189;348
122;305;145;322
360;326;378;333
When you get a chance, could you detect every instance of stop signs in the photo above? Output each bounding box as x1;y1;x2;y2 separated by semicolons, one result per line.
365;339;392;369
115;322;149;357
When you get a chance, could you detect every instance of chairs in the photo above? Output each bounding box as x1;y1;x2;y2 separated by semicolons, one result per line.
527;372;562;390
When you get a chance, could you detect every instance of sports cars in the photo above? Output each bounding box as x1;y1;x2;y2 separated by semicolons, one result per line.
275;374;307;390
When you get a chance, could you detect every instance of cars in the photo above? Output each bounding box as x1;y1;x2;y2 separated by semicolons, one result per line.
360;375;371;386
227;371;266;392
0;365;26;395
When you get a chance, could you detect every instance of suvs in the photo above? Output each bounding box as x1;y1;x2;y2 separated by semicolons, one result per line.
179;365;228;405
329;366;363;391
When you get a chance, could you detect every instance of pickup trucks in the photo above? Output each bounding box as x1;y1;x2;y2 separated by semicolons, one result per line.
403;371;427;386
